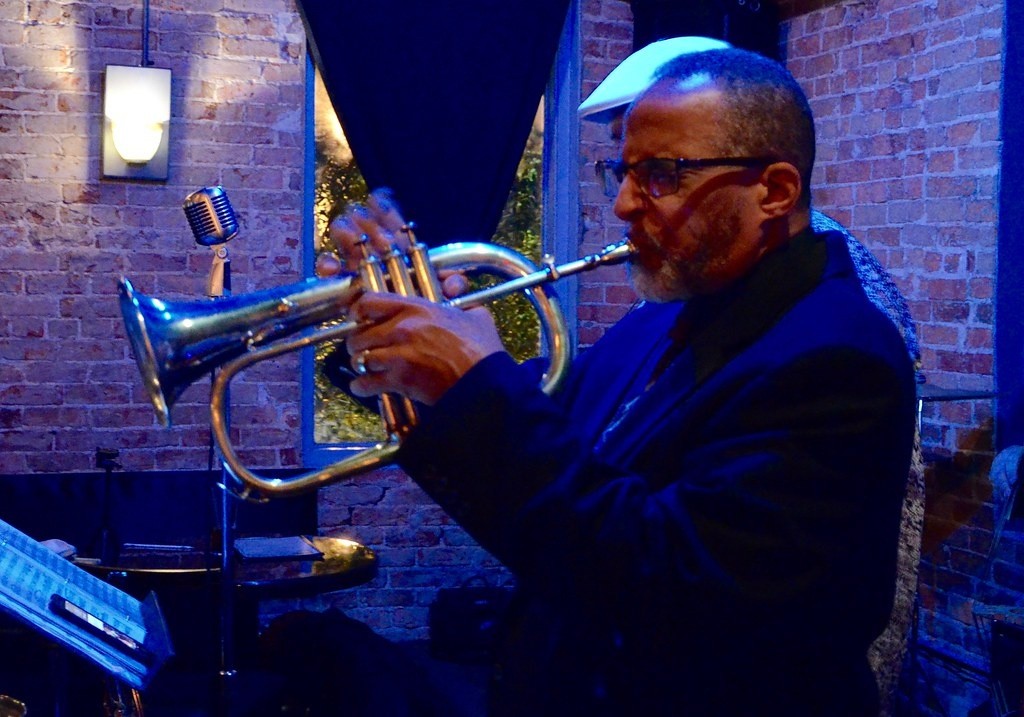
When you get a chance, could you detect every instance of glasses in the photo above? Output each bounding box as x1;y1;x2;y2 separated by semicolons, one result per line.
595;157;774;197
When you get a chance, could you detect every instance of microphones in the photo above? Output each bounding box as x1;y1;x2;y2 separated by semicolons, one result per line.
181;187;240;299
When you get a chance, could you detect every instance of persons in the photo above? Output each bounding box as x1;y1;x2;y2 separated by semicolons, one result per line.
317;35;930;717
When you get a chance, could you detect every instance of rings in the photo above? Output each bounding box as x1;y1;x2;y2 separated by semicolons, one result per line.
356;352;372;375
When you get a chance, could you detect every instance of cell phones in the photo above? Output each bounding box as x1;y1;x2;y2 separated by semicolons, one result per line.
48;594;151;666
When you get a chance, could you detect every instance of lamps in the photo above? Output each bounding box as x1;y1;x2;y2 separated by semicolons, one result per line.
100;63;173;184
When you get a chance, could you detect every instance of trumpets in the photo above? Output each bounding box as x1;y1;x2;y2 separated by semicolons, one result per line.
113;236;640;497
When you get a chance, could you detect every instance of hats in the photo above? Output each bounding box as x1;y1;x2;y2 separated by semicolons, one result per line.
577;36;735;124
